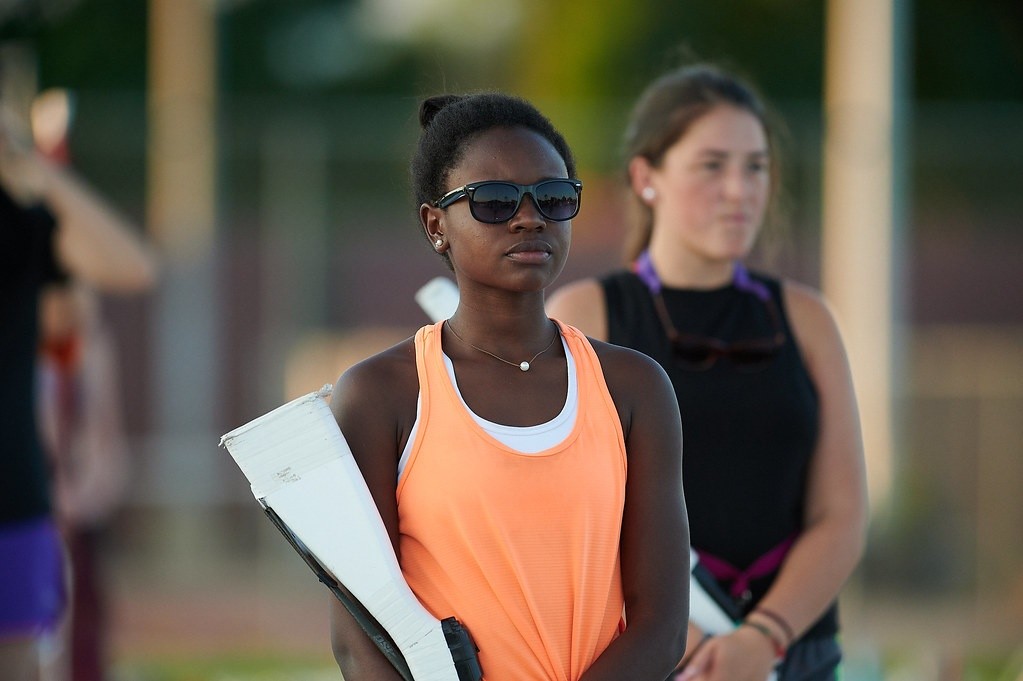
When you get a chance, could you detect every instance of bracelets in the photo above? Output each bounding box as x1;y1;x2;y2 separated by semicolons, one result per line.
743;621;783;661
753;607;796;645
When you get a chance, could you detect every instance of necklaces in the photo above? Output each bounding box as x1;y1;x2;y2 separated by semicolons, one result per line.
447;319;558;371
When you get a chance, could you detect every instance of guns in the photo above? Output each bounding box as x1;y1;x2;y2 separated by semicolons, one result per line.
224;390;485;681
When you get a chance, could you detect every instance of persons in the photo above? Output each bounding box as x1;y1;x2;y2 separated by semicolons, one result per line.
543;65;870;681
0;80;156;680
328;95;689;681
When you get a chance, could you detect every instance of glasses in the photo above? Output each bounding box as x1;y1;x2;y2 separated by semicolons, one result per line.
432;179;584;226
